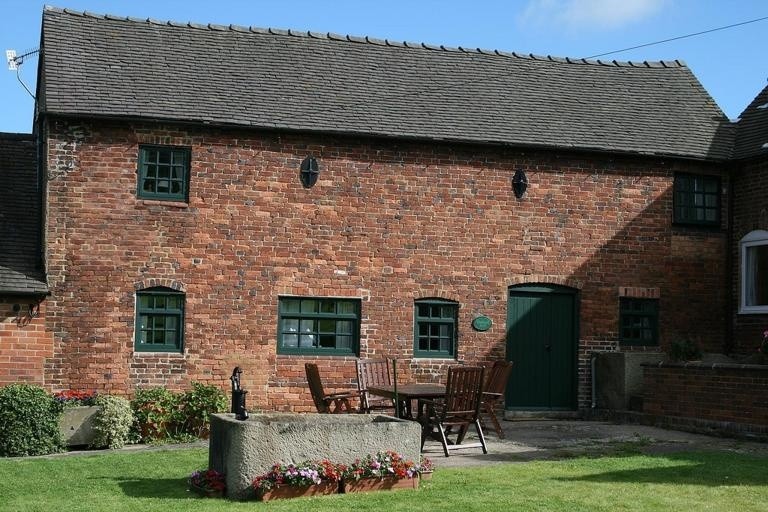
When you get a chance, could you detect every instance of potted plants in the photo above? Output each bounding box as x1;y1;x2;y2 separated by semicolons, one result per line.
0;380;231;458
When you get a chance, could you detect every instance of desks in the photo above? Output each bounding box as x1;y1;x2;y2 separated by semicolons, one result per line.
366;382;469;446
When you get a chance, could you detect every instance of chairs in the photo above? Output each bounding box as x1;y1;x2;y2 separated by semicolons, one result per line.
354;356;396;416
445;359;514;440
305;362;364;414
420;365;488;458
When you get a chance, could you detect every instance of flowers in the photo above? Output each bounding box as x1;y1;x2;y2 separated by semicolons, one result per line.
417;455;433;472
336;449;418;483
246;457;343;497
192;468;225;492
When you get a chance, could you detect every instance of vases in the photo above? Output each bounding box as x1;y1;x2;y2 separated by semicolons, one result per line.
419;470;434;481
254;481;340;502
206;491;224;500
341;478;419;494
